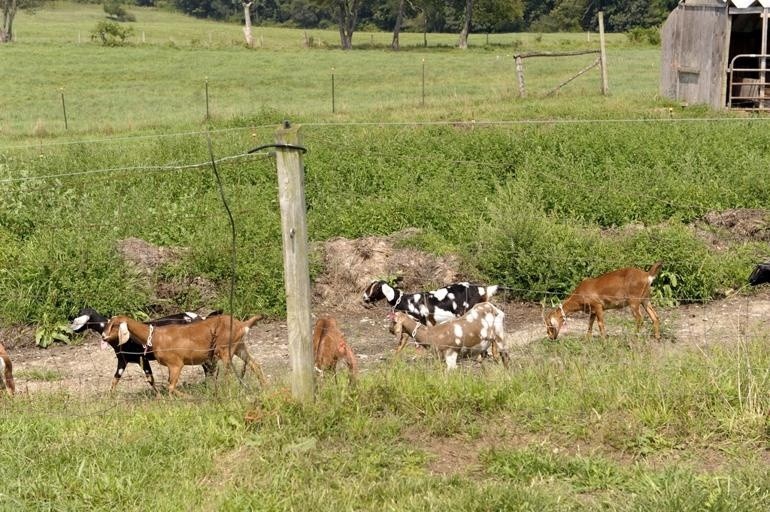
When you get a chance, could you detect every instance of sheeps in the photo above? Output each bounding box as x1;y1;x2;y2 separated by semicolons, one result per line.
362;279;498;367
312;316;358;386
0;343;15;401
102;313;268;402
748;263;770;286
543;261;664;343
71;308;224;398
388;301;511;370
